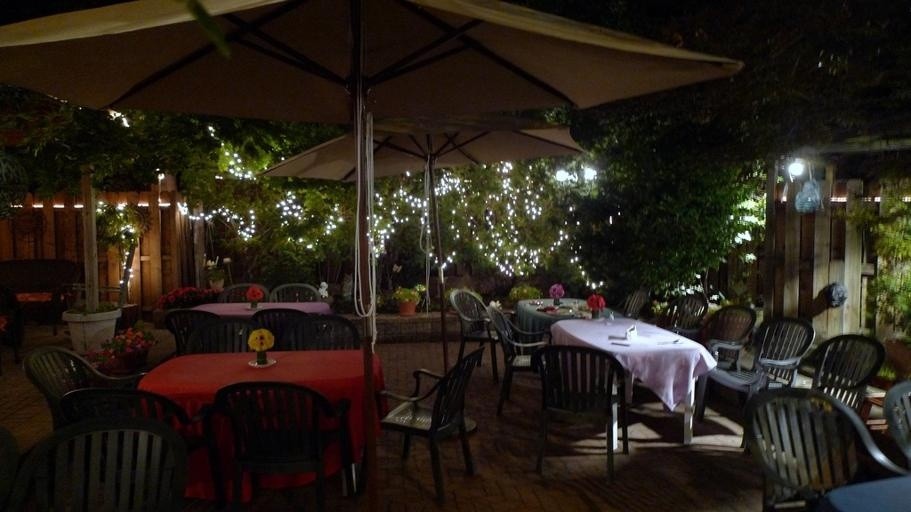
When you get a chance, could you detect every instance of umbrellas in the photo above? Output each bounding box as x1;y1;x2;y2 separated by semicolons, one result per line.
260;112;587;373
0;0;746;350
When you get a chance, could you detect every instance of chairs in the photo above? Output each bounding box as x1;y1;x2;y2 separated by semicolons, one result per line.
451;284;816;484
744;333;911;510
376;345;486;511
16;284;386;511
